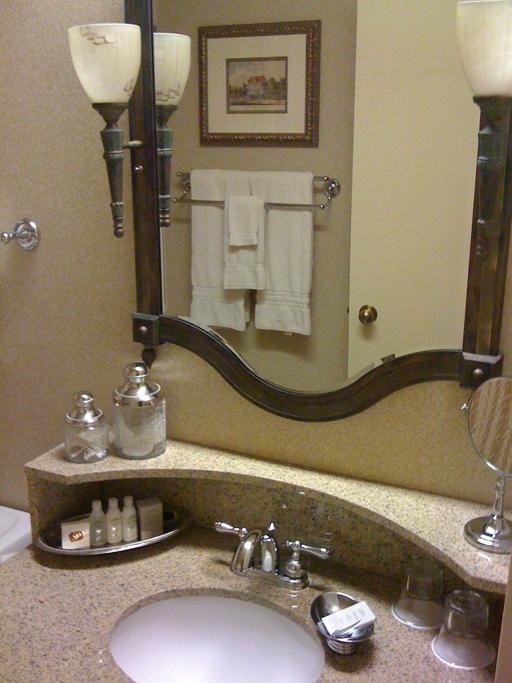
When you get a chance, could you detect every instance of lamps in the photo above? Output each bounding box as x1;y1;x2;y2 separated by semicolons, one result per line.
453;0;512;260
154;32;193;227
66;24;143;236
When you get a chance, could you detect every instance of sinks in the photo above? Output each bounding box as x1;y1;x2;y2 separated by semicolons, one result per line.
107;587;325;683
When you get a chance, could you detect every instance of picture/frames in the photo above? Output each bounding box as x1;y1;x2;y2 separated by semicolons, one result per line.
197;19;320;148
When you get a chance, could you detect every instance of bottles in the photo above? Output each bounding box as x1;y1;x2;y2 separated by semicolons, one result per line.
63;389;109;463
111;361;167;460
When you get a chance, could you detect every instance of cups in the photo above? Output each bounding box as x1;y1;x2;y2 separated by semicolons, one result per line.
431;590;497;671
392;560;446;630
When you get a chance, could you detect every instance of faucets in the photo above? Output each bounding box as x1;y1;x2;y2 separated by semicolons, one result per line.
229;530;264;577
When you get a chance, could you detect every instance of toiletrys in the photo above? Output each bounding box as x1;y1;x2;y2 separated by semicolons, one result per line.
89;499;108;547
106;497;122;544
121;495;138;542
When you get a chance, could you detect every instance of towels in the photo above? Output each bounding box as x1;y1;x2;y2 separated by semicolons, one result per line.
187;166;314;335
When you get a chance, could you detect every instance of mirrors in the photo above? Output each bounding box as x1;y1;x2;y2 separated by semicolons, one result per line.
122;0;512;422
461;376;512;556
